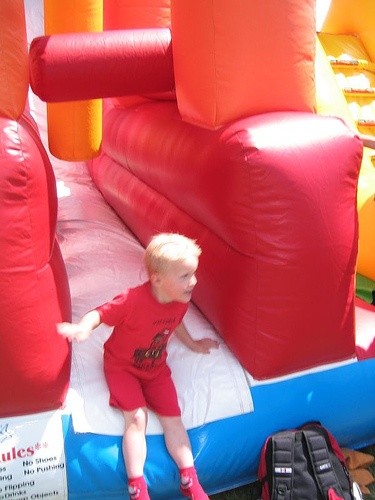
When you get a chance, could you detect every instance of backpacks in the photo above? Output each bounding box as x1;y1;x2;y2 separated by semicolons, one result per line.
259;420;362;500
